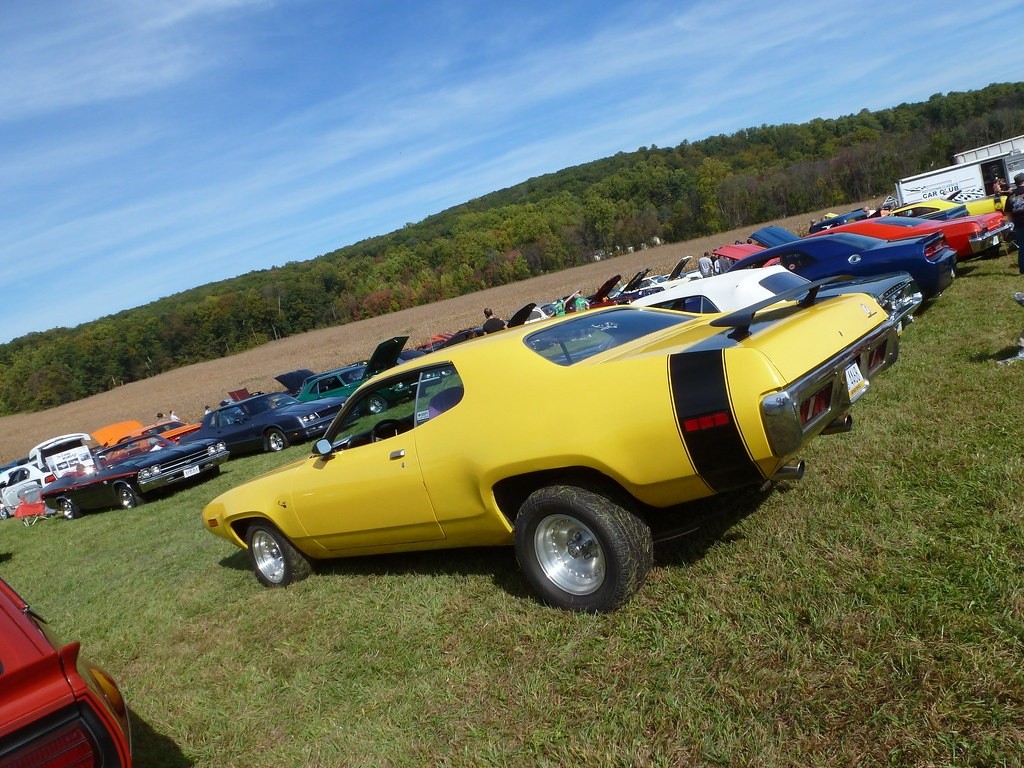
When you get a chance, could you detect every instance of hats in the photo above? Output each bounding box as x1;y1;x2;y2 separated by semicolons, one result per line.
1010;173;1024;184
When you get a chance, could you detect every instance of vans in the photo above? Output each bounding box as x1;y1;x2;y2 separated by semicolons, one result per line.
276;368;347;398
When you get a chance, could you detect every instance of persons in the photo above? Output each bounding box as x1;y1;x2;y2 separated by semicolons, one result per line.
483;308;508;335
808;219;817;234
698;252;714;278
993;178;1012;196
1004;173;1024;274
710;239;752;276
170;410;180;421
556;299;566;315
574;292;589;312
904;203;912;216
205;406;210;415
156;413;167;422
467;328;478;340
863;206;876;217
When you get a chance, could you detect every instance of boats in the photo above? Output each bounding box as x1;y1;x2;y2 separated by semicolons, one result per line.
728;232;958;304
825;191;1008;214
712;211;1017;262
628;264;924;335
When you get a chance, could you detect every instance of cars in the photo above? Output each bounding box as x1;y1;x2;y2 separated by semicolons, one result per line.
91;419;202;465
197;274;932;613
0;579;132;768
398;255;703;362
297;336;410;414
39;433;230;521
0;432;92;519
179;392;350;452
